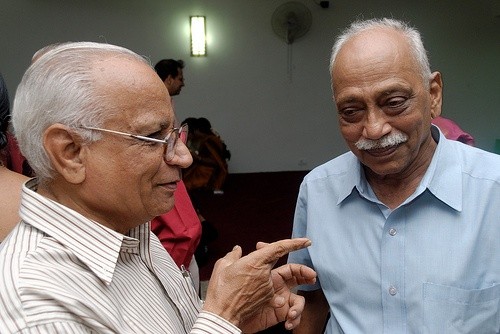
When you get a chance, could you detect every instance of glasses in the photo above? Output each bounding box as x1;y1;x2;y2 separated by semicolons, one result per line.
77;122;188;161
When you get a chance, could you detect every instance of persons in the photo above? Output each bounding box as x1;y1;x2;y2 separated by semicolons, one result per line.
1;40;318;334
153;57;185;96
285;14;500;334
0;74;36;245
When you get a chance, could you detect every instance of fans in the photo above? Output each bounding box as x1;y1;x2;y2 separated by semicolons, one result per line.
269;2;312;88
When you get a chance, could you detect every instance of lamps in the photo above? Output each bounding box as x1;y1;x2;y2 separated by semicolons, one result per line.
186;16;210;58
